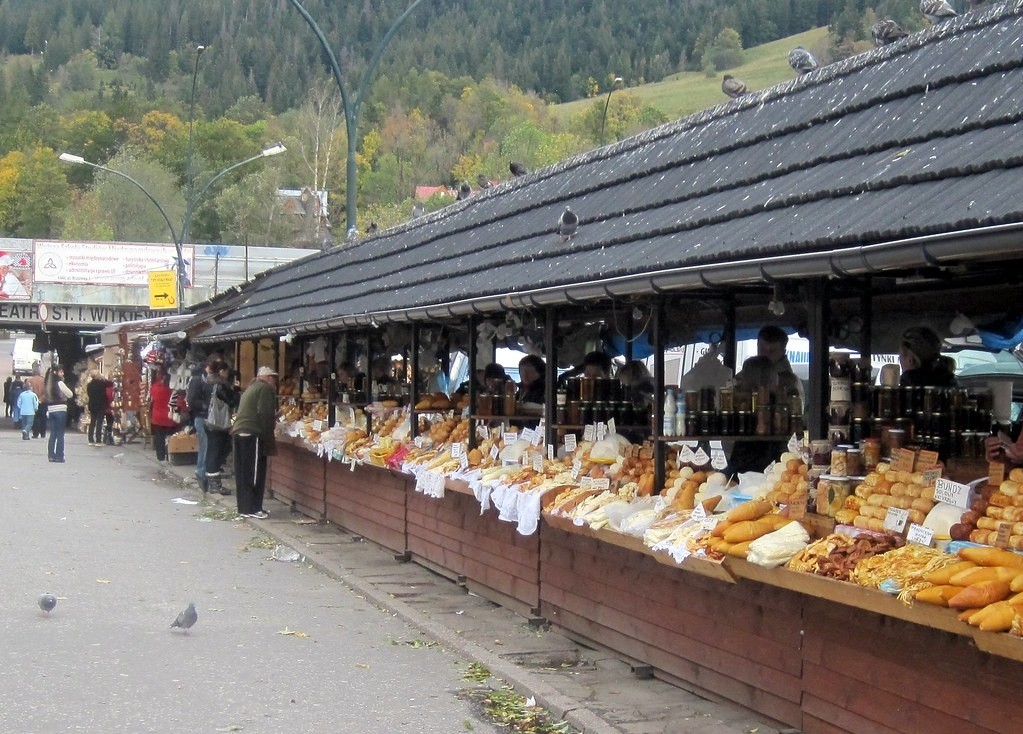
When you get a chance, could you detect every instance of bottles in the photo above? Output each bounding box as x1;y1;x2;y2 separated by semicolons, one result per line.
988;447;1009;487
662;388;686;437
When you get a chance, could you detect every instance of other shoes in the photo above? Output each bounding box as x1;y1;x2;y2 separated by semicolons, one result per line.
23;430;28;439
89;442;95;446
95;443;106;447
194;470;206;492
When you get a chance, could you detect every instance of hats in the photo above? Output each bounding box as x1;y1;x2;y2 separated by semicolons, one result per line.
259;367;279;376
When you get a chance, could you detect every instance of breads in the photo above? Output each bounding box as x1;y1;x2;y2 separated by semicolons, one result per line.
402;415;729;542
702;452;1023;638
415;393;470;410
277;402;469;461
279;378;322;399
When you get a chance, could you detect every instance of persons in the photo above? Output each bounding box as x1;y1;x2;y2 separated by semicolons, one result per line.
455;343;517;395
45;363;74;463
726;324;805;474
584;351;612;378
898;325;959;387
3;352;279;518
16;381;39;440
224;366;279;519
998;438;1023;464
618;361;654;386
515;354;546;405
281;357;405;407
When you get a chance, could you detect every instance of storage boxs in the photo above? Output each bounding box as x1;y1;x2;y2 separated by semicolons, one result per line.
168;435;200;453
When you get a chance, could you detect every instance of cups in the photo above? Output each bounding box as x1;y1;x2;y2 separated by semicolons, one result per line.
880;364;900;387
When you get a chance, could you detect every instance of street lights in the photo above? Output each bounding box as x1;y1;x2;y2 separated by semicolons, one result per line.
58;143;287;316
186;44;205;244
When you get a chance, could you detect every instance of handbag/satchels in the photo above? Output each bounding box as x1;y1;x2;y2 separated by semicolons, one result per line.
206;384;232;431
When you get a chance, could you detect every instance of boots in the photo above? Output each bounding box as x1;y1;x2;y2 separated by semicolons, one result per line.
205;471;232;494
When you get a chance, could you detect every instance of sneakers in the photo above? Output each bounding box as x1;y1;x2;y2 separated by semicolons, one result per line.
241;510;269;519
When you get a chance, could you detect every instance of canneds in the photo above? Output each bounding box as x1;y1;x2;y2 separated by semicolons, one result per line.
479;378;516;416
336;387;410;406
817;350;992;516
684;387;806;435
555;376;650;424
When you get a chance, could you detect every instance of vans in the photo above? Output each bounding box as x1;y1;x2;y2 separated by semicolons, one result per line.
9;338;43;376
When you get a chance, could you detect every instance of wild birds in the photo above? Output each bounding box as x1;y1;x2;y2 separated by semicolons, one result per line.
412;201;425;218
870;15;909;45
477;174;494;189
455;181;472;202
919;0;958;25
320;238;335;251
720;74;751;97
788;45;819;75
346;224;359;242
509;160;528;177
365;222;378;234
556;206;578;242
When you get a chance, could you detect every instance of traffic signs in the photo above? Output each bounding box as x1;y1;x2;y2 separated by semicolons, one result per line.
148;271;178;309
